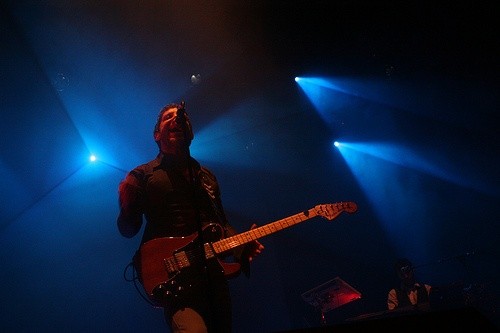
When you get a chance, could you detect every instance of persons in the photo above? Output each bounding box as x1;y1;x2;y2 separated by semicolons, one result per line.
387;258;434;311
116;104;264;333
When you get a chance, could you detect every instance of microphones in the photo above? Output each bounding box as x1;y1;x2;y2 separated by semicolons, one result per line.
400;265;415;272
174;100;186;128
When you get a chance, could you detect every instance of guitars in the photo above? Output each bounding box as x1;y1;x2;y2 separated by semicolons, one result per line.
137;198;359;308
